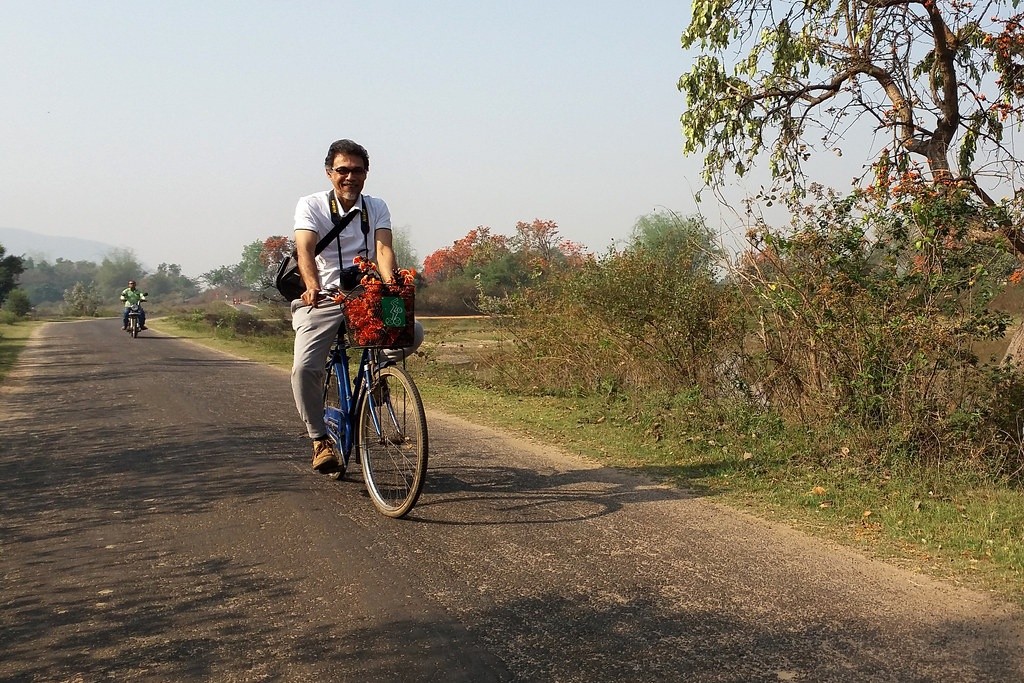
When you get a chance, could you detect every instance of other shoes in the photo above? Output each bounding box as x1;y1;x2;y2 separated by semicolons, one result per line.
141;326;148;330
121;326;127;330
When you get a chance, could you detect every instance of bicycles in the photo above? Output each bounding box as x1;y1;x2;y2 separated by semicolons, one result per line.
305;286;431;518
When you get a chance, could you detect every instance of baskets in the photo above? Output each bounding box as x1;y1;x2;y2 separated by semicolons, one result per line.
339;283;416;349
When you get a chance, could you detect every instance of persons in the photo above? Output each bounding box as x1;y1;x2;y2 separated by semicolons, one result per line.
216;292;240;305
120;280;148;331
290;139;425;471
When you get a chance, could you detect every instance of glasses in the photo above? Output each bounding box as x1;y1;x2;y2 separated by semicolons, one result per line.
128;283;135;284
331;166;366;175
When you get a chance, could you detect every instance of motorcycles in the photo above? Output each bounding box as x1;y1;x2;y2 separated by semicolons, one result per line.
120;292;149;339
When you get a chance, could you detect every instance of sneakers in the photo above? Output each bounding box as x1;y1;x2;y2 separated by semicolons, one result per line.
311;438;339;471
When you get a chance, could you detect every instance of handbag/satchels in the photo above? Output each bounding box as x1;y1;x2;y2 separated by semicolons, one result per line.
274;247;307;302
